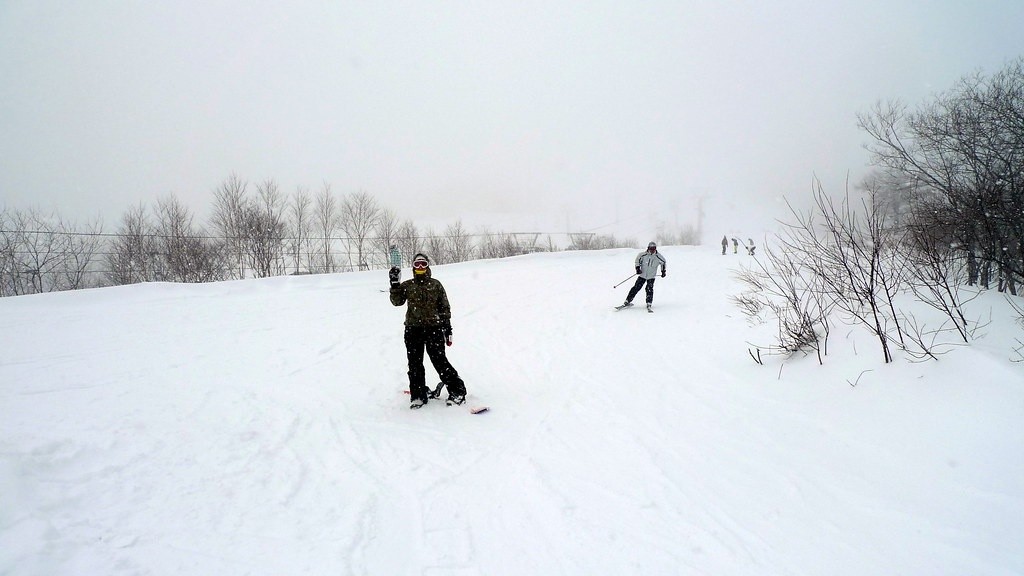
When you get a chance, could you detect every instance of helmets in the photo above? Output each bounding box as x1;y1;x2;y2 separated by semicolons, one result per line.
648;242;656;249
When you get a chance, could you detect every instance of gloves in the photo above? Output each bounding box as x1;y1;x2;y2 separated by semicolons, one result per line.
389;266;401;288
661;270;666;277
445;331;452;346
636;266;642;274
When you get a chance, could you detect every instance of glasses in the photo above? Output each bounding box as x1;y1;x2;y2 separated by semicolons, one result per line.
648;247;656;250
413;260;428;268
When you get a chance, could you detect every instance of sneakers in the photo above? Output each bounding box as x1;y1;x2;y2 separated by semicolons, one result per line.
622;301;628;306
647;303;652;310
410;398;423;410
446;394;466;406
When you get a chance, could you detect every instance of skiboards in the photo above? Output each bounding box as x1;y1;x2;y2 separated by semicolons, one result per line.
404;382;488;414
615;302;655;314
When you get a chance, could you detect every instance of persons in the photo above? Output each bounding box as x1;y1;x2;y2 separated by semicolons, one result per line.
722;236;728;255
732;238;738;254
389;253;467;410
746;239;756;255
622;241;666;308
